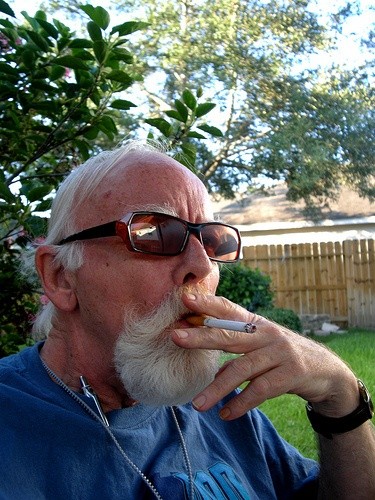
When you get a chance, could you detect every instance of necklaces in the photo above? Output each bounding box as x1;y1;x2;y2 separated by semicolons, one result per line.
38;354;194;500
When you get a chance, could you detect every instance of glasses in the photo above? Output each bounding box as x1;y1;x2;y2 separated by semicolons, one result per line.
57;211;241;263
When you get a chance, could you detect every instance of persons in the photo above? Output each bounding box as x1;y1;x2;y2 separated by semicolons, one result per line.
0;141;375;500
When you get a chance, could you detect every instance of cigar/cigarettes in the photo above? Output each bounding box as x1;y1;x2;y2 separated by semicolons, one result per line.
186;316;257;333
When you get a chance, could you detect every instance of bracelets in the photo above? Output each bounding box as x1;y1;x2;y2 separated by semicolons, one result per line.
306;379;374;440
